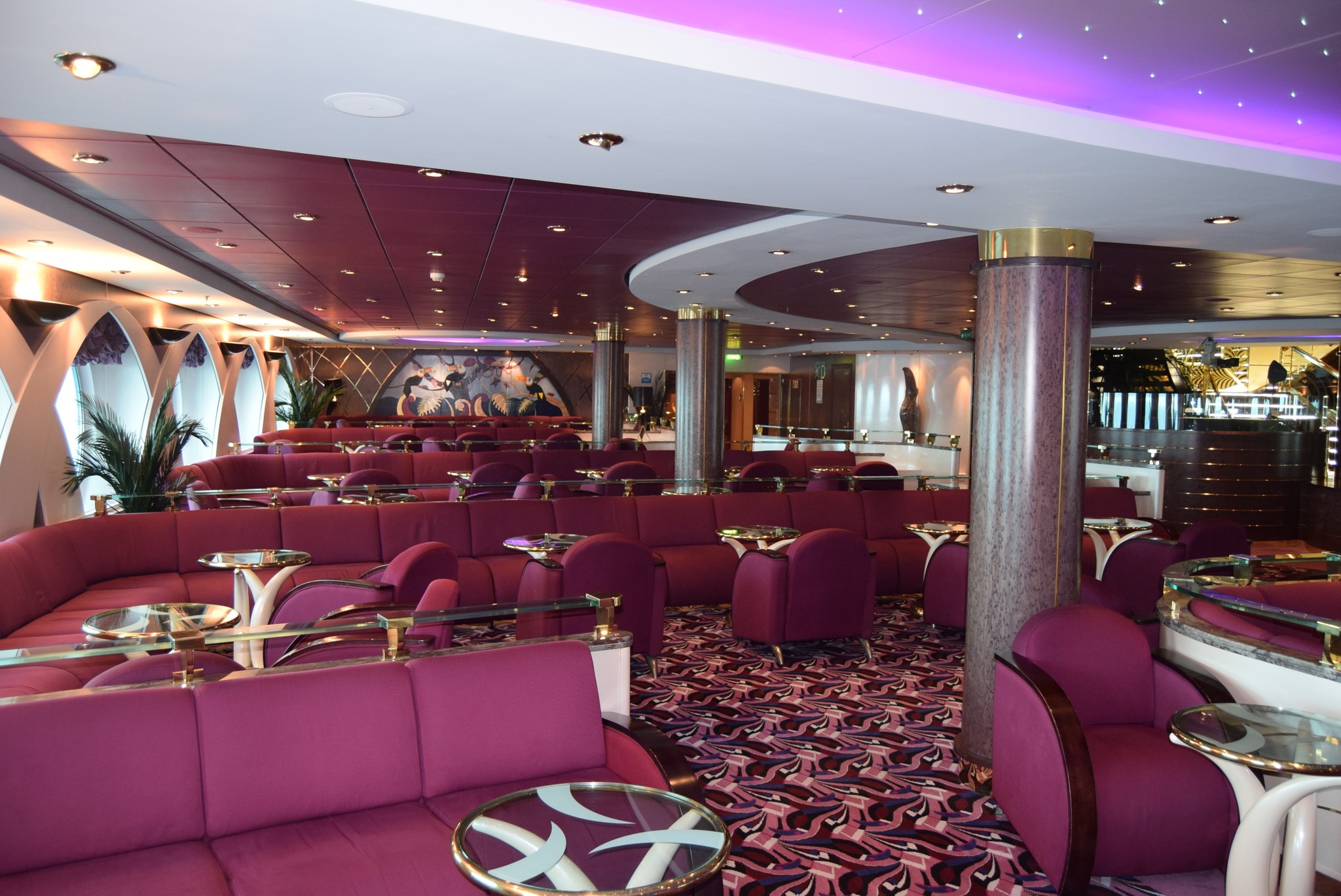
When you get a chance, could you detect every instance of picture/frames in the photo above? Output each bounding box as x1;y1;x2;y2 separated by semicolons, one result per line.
641;372;651;384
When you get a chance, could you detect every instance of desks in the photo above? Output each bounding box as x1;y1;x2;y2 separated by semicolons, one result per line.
1083;517;1154;582
661;486;733;495
307;472;353;487
715;525;802;558
809;464;854;474
81;603;242;661
1168;703;1341;896
574;467;609;480
447;470;473;480
336;493;418;505
1085;472;1132;487
448;778;732;896
503;533;587;559
902;518;970;581
197;549;312;669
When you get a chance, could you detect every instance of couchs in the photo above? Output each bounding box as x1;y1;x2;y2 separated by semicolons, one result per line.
0;641;726;896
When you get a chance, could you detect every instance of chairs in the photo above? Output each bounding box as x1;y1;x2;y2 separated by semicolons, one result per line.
921;541;969;642
186;481;268;510
991;602;1266;896
515;533;668;679
1101;517;1252;626
82;650;246;687
850;461;904;491
730;527;878;666
336;417;588;430
804;470;851;491
1083;486;1175;578
579;461;663;496
263;542;459;667
268;432;648;455
465;473;544;501
309;468;409;505
270;578;461;667
723;460;795;492
783;438;800;451
447;461;526;501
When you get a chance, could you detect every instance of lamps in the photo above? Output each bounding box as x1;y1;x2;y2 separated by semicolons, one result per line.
324;378;344;415
632;385;653;432
264;350;287;361
11;298;81;327
220;342;250;355
148;326;191;345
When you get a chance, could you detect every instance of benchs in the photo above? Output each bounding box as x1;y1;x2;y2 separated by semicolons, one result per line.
1189;581;1341;658
311;414;583;429
0;487;970;697
252;426;576;454
